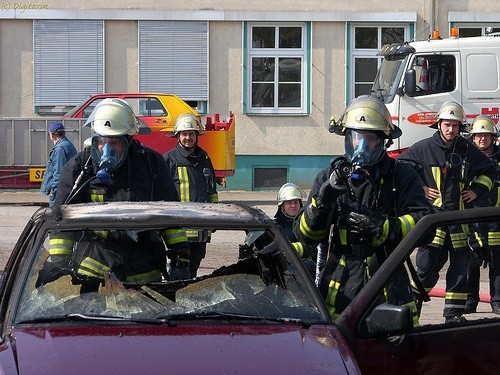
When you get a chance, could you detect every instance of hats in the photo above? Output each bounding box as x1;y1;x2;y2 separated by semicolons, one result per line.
46;122;65;132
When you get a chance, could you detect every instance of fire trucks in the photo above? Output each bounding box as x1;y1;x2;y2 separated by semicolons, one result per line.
370;26;500;159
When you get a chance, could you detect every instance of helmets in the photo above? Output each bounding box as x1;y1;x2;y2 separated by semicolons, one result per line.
340;99;392;132
471;117;495;134
90;98;139;135
438;106;464;123
174;116;201;136
277;186;303;202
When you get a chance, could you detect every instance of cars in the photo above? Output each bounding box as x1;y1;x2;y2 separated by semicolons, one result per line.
0;201;500;375
63;93;202;131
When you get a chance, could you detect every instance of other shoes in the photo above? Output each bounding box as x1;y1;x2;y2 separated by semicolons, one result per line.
462;302;476;314
493;305;500;314
445;315;467;323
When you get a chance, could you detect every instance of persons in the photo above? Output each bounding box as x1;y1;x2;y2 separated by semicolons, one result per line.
397;101;495;325
272;182;312;277
292;95;435;328
48;98;189;284
38;121;77;209
466;113;499;315
163;113;219;279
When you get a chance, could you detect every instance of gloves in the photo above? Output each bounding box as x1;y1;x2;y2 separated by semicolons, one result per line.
316;179;346;208
170;268;190;281
349;212;384;239
